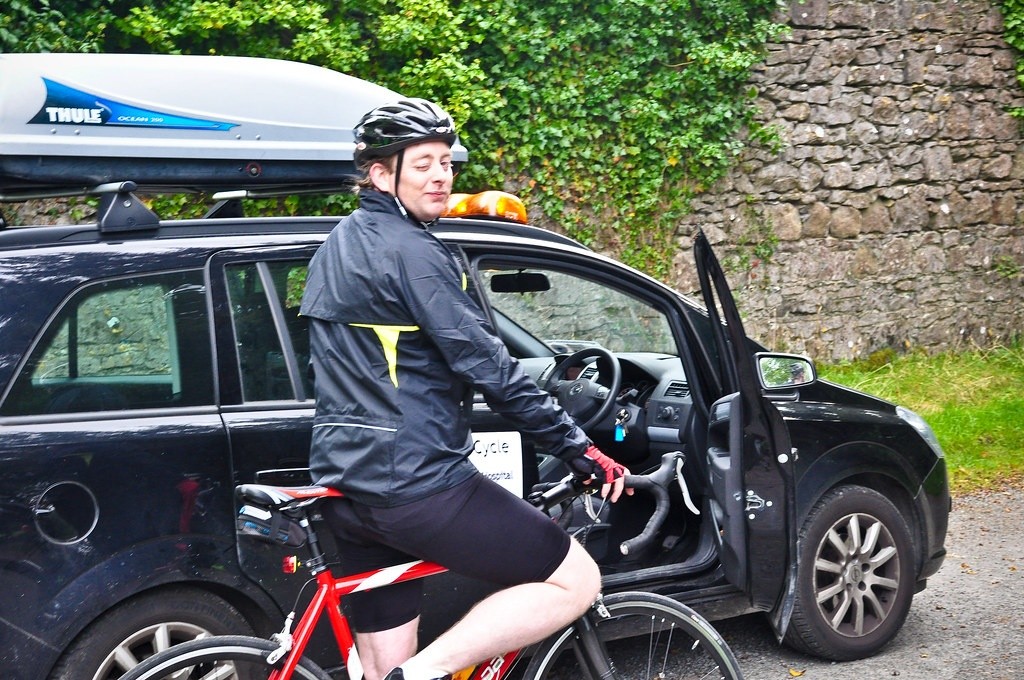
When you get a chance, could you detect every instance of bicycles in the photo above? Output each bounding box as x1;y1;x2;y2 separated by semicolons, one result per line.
117;451;747;680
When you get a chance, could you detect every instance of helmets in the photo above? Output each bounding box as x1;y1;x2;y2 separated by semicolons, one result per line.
354;98;455;167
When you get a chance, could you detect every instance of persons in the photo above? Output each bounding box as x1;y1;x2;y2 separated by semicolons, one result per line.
298;99;633;680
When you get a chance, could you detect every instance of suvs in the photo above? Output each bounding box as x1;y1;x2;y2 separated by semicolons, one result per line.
0;50;954;680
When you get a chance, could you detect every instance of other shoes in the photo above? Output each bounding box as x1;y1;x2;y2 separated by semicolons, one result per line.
383;667;451;680
